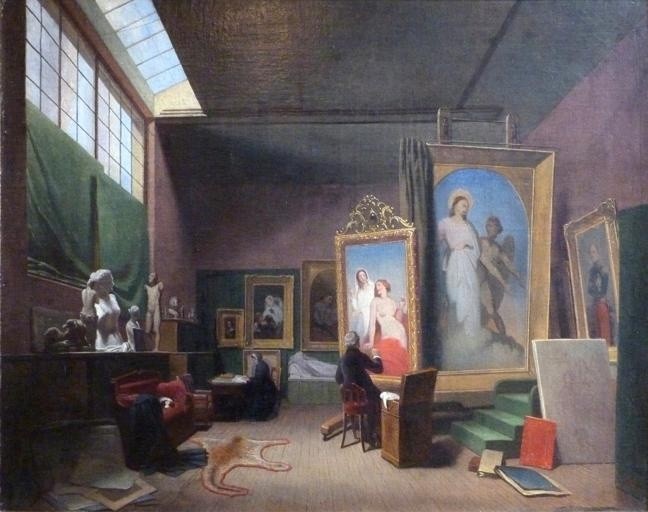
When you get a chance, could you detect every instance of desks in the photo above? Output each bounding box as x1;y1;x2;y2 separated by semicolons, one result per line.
206;375;251;421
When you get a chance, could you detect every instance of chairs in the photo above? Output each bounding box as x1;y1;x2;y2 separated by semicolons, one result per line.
269;367;279;390
338;383;379;451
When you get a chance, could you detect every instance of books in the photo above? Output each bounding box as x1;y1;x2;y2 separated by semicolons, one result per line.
518;415;559;469
477;448;504;478
467;455;506;473
494;465;574;497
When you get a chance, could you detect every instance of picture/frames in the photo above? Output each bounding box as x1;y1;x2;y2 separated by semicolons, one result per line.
563;198;621;364
217;308;244;348
242;349;282;379
244;274;295;349
300;259;339;352
424;144;556;407
334;194;419;392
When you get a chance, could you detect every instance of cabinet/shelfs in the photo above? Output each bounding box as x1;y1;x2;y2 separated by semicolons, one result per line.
159;319;214;387
379;368;439;466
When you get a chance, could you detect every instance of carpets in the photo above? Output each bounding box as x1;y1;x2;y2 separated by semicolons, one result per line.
192;423;290;497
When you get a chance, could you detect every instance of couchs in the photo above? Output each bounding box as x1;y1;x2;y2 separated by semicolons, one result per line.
185;374;213;430
111;369;194;447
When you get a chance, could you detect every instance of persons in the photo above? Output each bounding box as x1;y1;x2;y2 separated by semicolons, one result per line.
335;330;384;450
242;351;276;422
80;278;100;316
89;268;135;353
142;270;164;353
478;215;525;327
124;305;141;352
310;292;334;341
362;277;410;352
349;267;376;338
434;186;481;335
226;320;236;339
586;239;613;347
253;294;283;339
